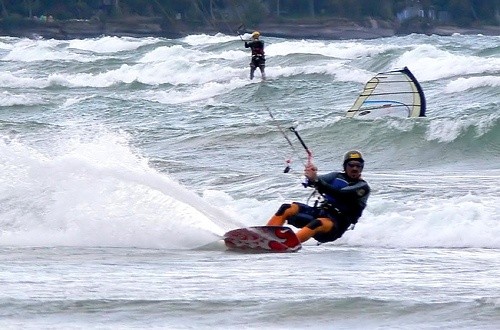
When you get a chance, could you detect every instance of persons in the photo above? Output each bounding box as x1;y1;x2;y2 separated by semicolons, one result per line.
265;151;371;245
244;32;266;80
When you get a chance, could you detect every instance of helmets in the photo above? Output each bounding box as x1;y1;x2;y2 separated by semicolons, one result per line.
344;151;364;163
252;31;260;38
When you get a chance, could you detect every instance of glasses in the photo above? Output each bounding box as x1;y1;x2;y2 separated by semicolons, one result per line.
348;163;361;168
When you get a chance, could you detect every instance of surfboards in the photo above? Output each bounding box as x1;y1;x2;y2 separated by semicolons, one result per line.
222;225;303;251
